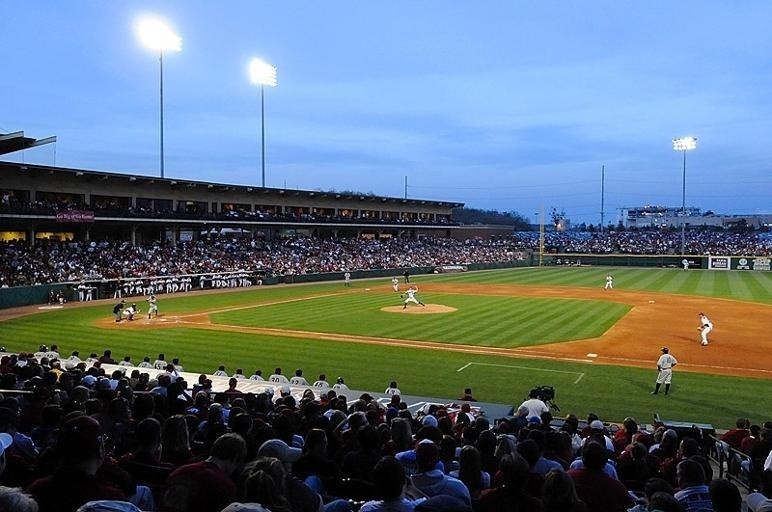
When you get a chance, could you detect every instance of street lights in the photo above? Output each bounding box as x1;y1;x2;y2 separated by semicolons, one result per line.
133;13;183;179
672;135;698;255
248;55;277;188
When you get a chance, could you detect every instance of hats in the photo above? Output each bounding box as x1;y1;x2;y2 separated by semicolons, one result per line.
257;438;303;461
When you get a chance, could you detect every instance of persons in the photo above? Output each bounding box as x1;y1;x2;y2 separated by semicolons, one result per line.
531;226;771;272
124;303;139;323
114;296;124;322
696;313;716;345
518;389;550;424
1;190;530;305
352;393;772;512
457;388;478;402
604;273;613;290
402;285;425;310
146;292;159;323
650;347;678;394
1;343;352;512
385;382;401;395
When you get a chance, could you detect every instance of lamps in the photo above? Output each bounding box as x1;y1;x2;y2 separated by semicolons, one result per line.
20;163;462;210
